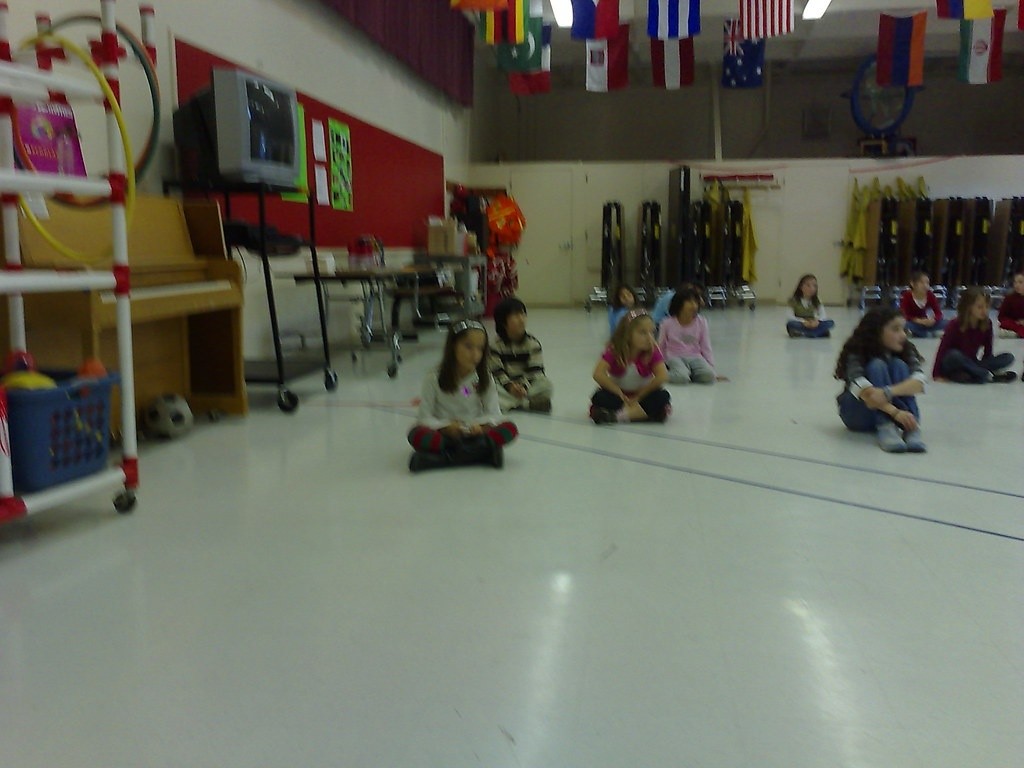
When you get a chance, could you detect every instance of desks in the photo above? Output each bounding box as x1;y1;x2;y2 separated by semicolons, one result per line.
293;265;465;383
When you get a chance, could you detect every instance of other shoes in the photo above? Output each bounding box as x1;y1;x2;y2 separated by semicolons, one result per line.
649;411;669;424
409;452;439;473
590;406;617;425
483;448;503;469
528;398;552;413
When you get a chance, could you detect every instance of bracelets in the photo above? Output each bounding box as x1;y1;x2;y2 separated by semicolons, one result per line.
892;408;900;418
883;385;896;401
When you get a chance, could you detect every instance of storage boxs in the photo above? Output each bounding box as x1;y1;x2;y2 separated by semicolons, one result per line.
420;215;458;256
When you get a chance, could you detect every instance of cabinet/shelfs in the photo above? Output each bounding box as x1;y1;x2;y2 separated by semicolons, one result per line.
0;0;147;522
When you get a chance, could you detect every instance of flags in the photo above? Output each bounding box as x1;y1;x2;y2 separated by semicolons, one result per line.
1017;0;1024;31
879;11;926;87
740;0;795;38
722;18;765;90
450;0;552;92
647;0;701;90
570;0;629;93
937;0;1007;84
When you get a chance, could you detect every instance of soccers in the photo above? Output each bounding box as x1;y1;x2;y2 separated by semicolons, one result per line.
144;395;194;435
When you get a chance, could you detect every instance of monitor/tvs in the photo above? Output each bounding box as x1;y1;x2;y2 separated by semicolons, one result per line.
173;65;300;196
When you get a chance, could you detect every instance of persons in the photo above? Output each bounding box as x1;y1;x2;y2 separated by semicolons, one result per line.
900;271;953;338
590;309;673;423
998;274;1024;339
608;284;654;336
407;320;518;473
786;274;835;338
836;309;930;453
488;298;553;413
657;289;730;383
933;288;1018;384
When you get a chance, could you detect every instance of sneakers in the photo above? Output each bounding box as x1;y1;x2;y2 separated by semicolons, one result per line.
992;370;1018;382
904;428;927;452
877;425;907;452
